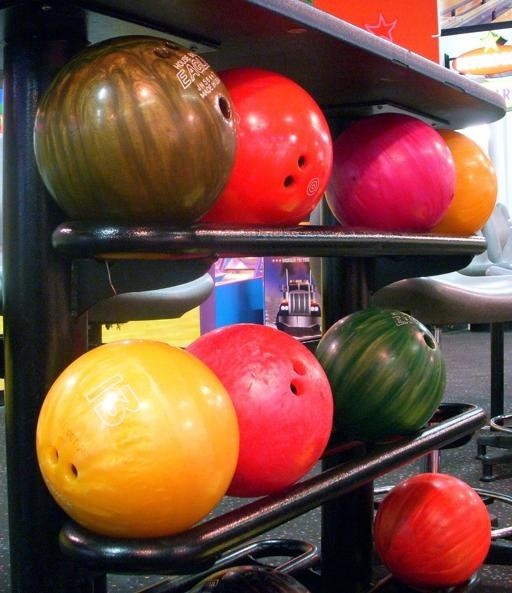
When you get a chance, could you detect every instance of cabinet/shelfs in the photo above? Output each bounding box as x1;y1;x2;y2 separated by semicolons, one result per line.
2;0;511;591
